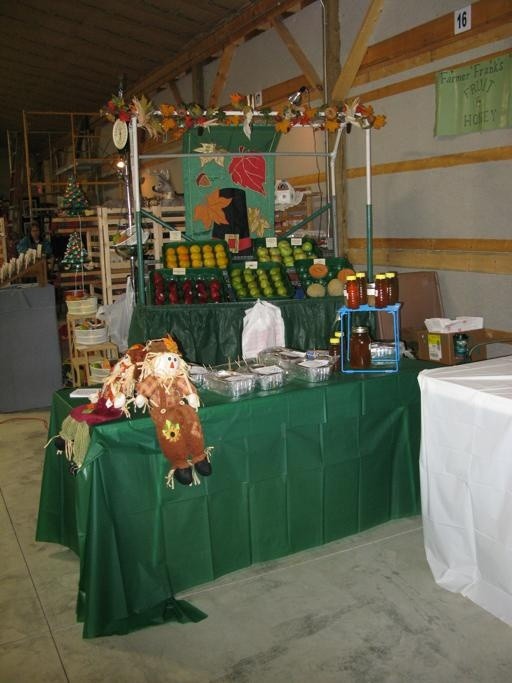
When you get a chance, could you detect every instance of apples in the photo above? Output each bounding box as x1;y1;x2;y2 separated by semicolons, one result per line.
258;239;318;267
153;272;221;305
228;268;287;296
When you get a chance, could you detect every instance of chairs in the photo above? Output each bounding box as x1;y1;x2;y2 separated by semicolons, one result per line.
67;313;119;388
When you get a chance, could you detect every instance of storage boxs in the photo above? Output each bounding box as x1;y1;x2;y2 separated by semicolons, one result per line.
417;327;486;365
148;238;355;304
375;271;444;340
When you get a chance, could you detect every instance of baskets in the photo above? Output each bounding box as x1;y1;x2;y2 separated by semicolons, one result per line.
146;237;357;308
65;295;120;384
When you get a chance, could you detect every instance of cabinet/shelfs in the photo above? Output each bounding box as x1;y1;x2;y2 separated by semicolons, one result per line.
125;100;378;366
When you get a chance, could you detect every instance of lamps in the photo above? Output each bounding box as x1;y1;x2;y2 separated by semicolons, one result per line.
287;86;305;105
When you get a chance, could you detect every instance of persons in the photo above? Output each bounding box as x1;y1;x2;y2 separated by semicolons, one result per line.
15;219;55;284
136;333;218;491
46;342;147;465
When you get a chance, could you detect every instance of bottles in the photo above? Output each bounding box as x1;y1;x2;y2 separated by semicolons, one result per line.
385;272;399;305
356;272;368;305
375;274;387;308
329;339;340;367
344;275;359;308
367;283;376;307
334;331;346;363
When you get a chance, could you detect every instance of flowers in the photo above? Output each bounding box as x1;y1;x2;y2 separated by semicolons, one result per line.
99;93;386;143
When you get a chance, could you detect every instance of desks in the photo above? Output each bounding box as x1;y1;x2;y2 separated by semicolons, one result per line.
0;284;64;413
416;356;511;628
34;358;449;639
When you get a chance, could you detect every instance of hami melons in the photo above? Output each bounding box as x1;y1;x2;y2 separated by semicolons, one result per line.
306;264;356;298
